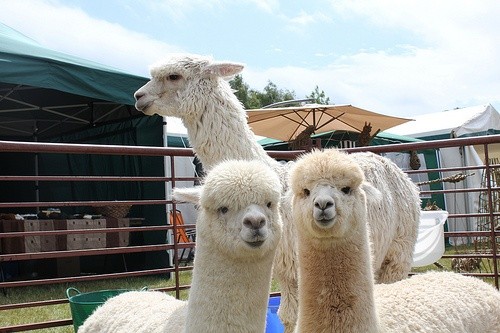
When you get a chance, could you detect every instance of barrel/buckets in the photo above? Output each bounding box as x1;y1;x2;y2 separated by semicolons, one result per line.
66;287;147;333
265;297;284;333
